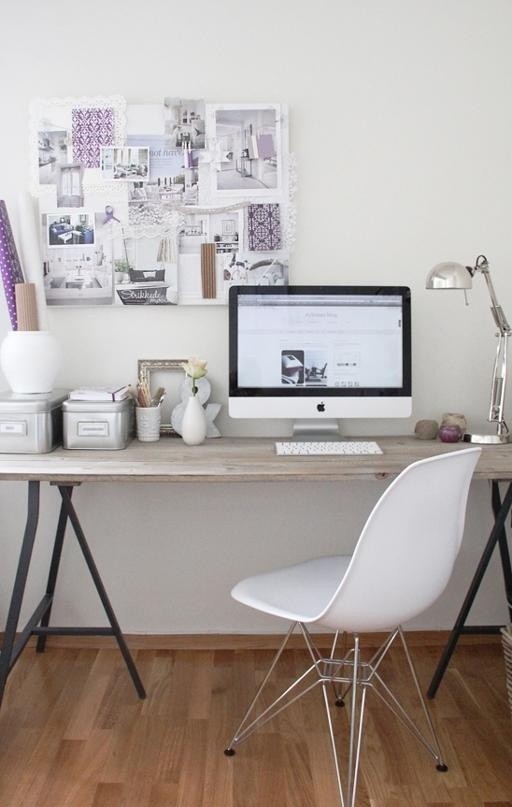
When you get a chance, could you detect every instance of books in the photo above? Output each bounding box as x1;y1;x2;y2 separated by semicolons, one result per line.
68;386;129;401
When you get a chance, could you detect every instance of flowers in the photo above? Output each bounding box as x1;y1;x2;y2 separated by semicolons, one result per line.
181;358;207;396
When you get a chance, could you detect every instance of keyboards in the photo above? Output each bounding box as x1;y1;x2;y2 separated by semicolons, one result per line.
275;442;383;456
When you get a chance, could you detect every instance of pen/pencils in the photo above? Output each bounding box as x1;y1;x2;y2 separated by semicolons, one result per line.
128;376;164;406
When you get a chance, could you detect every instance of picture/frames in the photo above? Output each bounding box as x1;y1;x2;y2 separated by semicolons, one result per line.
25;95;129;196
138;359;193;438
204;103;282;197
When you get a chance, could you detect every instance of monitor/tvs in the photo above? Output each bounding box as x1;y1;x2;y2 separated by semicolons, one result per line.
228;286;413;435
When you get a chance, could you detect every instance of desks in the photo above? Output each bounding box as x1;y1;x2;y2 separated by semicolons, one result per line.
0;436;512;709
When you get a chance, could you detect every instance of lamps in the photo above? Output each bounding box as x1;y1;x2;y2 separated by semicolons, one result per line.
425;254;511;445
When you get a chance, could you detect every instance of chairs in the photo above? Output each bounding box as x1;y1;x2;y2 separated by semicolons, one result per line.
223;447;482;807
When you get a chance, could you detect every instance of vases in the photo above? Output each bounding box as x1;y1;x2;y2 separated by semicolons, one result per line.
181;396;208;446
0;331;62;394
